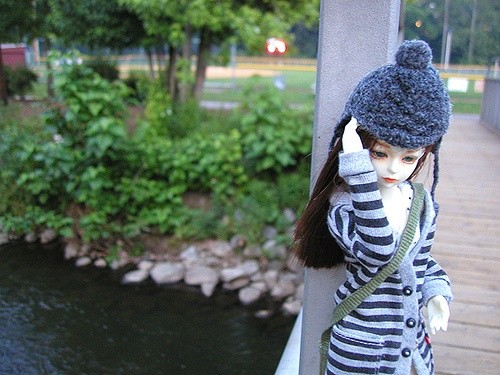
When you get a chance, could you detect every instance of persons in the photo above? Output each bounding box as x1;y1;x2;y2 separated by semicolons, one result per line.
287;40;453;374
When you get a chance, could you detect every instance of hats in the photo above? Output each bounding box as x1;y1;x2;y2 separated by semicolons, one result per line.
326;39;452;201
